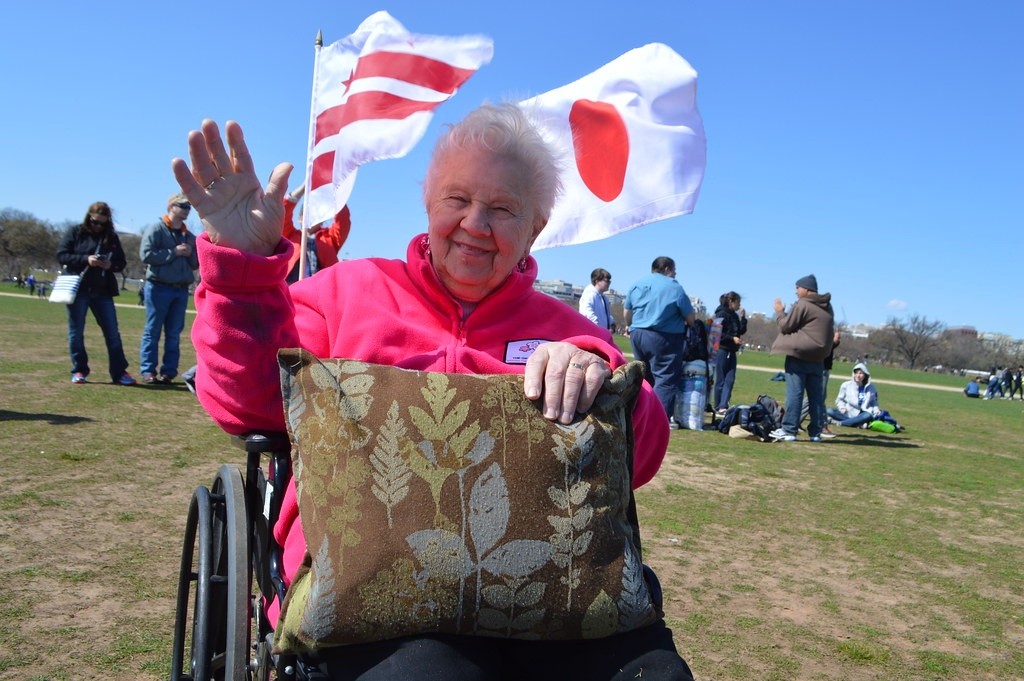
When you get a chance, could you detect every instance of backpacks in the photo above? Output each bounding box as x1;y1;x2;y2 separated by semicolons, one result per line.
681;319;711;361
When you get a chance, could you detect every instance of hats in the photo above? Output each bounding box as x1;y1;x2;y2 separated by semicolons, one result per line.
795;274;817;292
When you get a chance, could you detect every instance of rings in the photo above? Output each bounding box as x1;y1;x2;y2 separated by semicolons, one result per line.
587;359;603;368
568;363;584;370
205;181;214;189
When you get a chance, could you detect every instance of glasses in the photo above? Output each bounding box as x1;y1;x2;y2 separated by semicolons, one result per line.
90;217;110;228
601;278;611;284
173;203;192;209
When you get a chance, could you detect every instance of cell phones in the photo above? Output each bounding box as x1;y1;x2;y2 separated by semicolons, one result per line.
96;255;107;260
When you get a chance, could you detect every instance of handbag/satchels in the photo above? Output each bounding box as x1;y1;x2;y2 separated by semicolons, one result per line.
48;274;82;305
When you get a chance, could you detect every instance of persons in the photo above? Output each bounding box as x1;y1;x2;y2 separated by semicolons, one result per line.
624;257;694;428
171;105;693;681
17;274;48;297
579;268;618;334
925;366;927;372
713;292;748;416
766;274;834;441
57;202;136;385
855;352;868;365
964;364;1024;401
138;287;144;305
799;332;840;437
827;363;882;429
282;184;350;284
141;194;198;384
949;367;964;376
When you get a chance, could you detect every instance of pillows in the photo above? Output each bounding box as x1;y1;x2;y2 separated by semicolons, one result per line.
272;348;663;656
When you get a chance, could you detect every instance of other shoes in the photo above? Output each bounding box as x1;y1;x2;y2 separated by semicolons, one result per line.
809;435;822;441
768;428;796;441
143;373;156;383
715;409;727;417
160;374;172;385
859;422;867;429
827;416;835;424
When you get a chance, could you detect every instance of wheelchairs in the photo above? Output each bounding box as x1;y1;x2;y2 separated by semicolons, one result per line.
168;431;666;681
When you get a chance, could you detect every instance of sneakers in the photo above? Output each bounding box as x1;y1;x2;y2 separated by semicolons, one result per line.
819;427;837;438
117;372;137;385
71;372;86;383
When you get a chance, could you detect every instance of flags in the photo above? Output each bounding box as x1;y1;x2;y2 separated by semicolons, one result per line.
518;43;706;253
303;10;494;230
